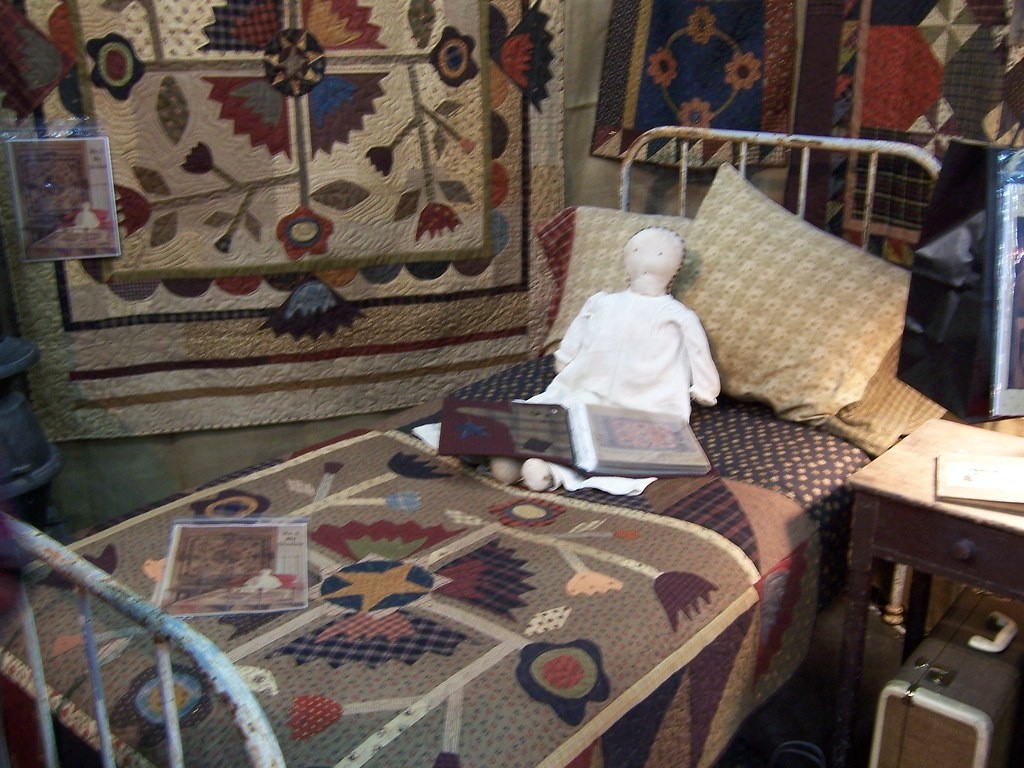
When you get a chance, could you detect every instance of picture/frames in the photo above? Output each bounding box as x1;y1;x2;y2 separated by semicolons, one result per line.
989;183;1024;420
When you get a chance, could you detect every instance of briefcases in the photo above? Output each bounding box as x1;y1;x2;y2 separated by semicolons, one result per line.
869;582;1024;767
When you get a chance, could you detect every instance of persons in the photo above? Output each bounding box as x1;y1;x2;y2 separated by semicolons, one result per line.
492;223;723;497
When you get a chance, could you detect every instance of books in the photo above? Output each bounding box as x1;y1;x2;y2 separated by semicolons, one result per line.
438;394;713;477
935;452;1024;515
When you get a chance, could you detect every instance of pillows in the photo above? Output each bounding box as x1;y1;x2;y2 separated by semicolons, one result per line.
540;159;950;456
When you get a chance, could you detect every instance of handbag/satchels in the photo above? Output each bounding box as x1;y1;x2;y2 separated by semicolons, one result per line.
896;130;1023;423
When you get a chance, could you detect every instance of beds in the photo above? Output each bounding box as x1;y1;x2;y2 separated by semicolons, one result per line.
0;125;943;768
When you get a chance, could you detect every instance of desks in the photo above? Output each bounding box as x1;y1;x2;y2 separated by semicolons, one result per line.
831;416;1024;768
